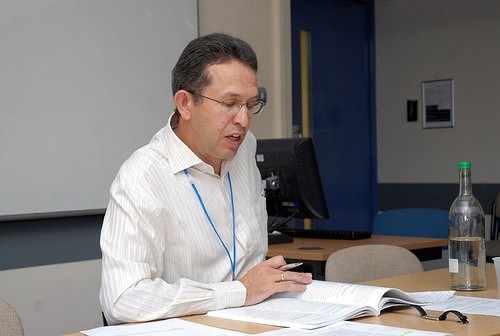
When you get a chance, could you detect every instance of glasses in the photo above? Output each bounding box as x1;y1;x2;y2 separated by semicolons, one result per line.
188;90;265;115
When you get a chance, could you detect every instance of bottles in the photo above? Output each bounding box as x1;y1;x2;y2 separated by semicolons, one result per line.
448;161;489;291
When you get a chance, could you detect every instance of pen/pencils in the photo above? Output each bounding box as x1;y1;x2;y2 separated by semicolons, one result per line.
277;262;304;271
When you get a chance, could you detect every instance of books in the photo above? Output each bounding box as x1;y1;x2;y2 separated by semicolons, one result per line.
206;280;426;330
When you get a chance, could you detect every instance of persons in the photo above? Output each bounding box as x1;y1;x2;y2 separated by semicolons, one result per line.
98;32;313;325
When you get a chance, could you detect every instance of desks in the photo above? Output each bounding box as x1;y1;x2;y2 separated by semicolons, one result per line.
265;234;448;281
62;261;500;336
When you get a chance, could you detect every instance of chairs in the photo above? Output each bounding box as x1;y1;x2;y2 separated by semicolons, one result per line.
483;193;500;262
1;258;104;336
371;208;450;239
325;244;424;283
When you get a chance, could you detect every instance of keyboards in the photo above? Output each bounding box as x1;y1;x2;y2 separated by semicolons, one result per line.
283;229;374;241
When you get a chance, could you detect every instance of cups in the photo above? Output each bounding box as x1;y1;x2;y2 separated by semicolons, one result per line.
491;256;500;292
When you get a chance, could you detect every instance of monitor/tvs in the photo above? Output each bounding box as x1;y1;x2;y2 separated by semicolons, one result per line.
253;138;329;245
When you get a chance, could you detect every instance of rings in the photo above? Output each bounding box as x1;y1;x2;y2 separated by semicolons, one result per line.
281;273;285;281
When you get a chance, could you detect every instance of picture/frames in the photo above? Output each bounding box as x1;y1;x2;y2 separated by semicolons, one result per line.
421;78;455;128
